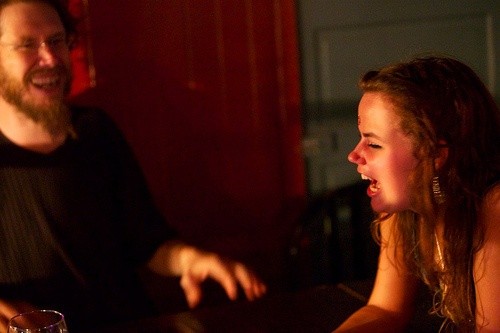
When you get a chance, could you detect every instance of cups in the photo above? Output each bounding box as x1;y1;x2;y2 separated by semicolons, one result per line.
8;310;68;333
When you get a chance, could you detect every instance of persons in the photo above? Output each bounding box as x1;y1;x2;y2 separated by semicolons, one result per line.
330;52;500;333
0;0;268;333
